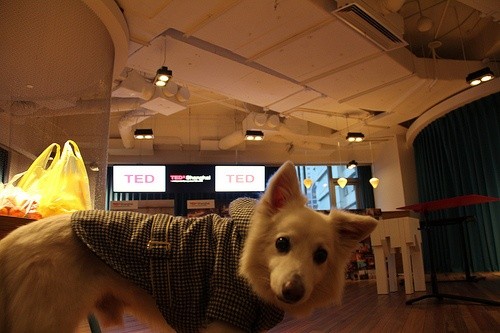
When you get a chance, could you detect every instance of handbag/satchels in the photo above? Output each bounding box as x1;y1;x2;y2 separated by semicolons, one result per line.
16;141;91;220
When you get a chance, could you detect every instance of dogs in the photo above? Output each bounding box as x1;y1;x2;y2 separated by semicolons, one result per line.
0;160;379;332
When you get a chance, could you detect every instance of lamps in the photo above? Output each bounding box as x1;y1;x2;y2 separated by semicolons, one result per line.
455;6;495;87
348;159;359;170
337;132;348;188
368;141;379;189
153;36;176;88
303;141;313;189
346;118;363;140
133;99;157;140
245;113;264;141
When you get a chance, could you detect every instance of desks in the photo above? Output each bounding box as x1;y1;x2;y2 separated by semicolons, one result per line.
396;193;500;307
370;216;425;296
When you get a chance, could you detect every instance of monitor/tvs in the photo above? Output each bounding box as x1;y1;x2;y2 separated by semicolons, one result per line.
111;163;167;193
213;165;266;193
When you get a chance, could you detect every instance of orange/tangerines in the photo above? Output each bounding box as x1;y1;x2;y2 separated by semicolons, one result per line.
0;206;42;220
